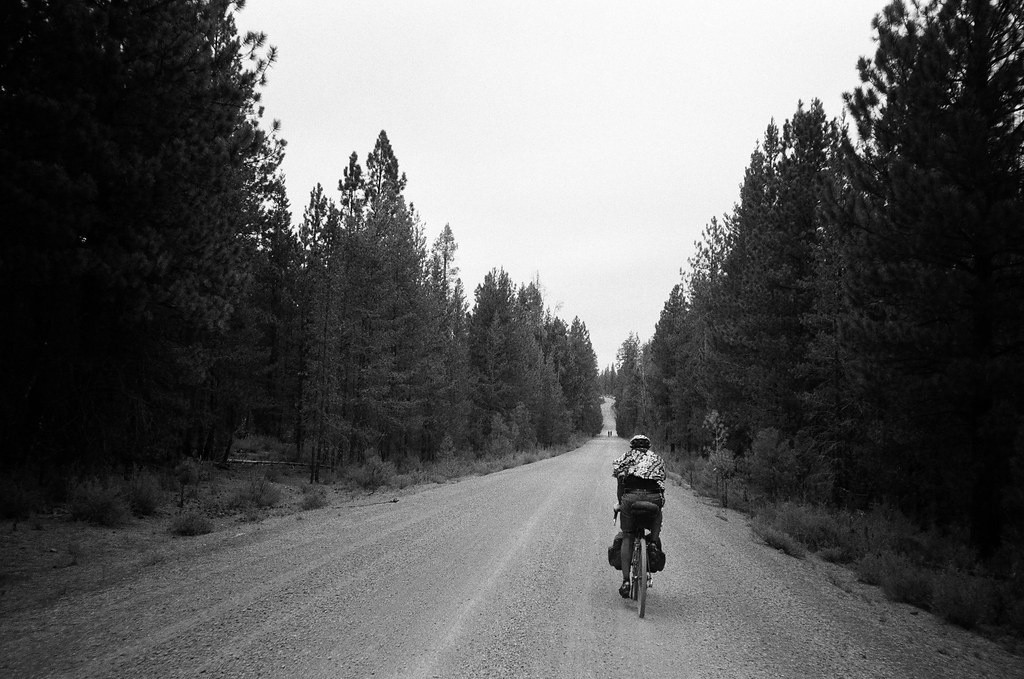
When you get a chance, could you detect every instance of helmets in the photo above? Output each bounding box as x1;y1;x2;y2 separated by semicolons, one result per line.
630;435;651;449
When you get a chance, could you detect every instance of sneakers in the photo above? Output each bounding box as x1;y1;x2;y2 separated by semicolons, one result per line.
620;580;630;594
648;543;658;555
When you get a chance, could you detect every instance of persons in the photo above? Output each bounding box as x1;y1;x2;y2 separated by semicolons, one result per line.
611;434;667;597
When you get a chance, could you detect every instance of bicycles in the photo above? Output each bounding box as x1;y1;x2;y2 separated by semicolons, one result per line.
612;500;658;618
